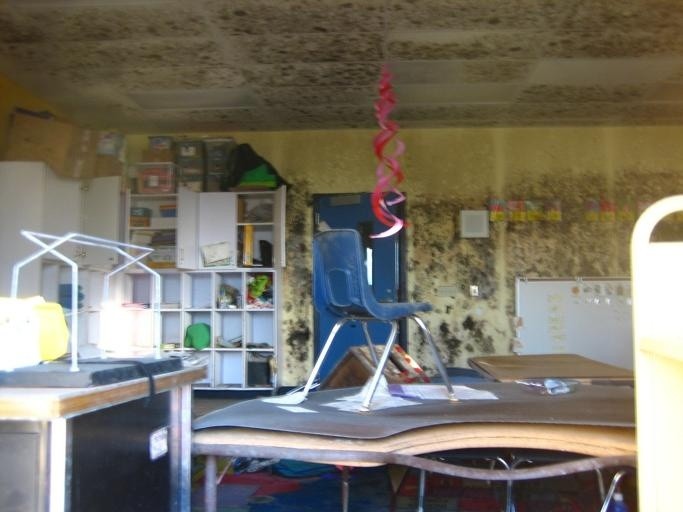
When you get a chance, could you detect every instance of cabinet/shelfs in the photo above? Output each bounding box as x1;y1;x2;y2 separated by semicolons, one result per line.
122;185;283;397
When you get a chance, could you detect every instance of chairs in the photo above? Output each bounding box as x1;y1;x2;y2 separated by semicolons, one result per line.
303;227;458;411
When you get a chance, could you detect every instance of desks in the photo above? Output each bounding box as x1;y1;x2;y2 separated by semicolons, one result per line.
467;354;633;388
190;382;637;512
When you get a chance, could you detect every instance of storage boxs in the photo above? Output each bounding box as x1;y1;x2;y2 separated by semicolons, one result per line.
131;136;237;192
